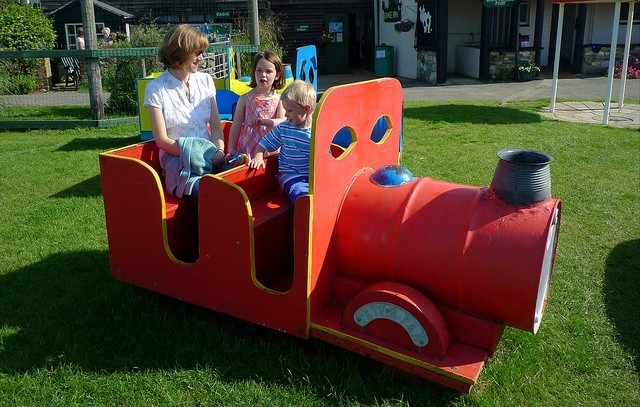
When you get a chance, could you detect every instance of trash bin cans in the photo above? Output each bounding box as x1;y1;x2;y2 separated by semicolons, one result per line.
375;45;393;77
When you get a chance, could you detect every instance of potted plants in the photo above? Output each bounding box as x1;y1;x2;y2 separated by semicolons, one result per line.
515;59;541;80
395;19;415;32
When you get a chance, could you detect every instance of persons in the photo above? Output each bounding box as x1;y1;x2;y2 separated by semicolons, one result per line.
98;28;111;43
228;51;286;157
74;28;84;79
247;80;316;213
144;24;224;260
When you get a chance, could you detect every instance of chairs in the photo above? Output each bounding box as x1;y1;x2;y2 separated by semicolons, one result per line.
60;56;80;90
